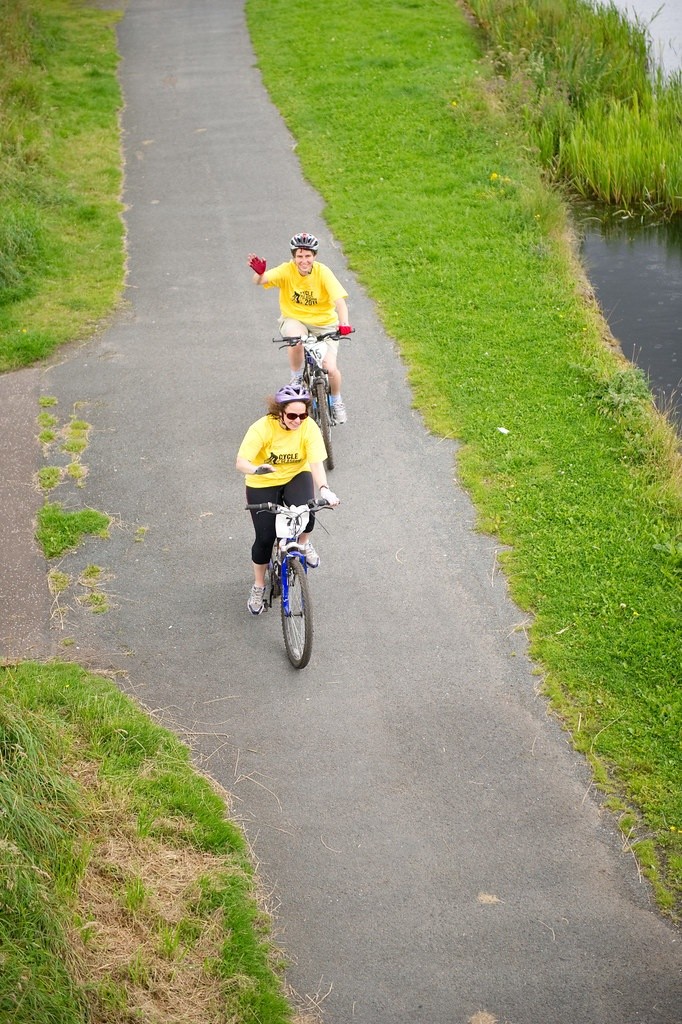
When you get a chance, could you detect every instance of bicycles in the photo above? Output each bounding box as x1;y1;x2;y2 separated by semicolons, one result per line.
244;499;342;669
272;326;355;471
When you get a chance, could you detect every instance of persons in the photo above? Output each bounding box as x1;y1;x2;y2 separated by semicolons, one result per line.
247;233;352;425
236;385;339;616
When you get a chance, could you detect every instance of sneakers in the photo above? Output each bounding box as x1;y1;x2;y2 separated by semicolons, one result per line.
332;396;347;425
297;536;320;568
289;370;304;386
247;584;266;617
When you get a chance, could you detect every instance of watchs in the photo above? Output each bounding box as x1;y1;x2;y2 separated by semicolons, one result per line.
319;485;329;491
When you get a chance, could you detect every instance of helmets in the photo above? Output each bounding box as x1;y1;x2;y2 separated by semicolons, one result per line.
275;383;311;403
289;232;319;251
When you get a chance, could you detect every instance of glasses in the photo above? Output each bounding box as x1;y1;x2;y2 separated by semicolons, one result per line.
283;411;309;419
